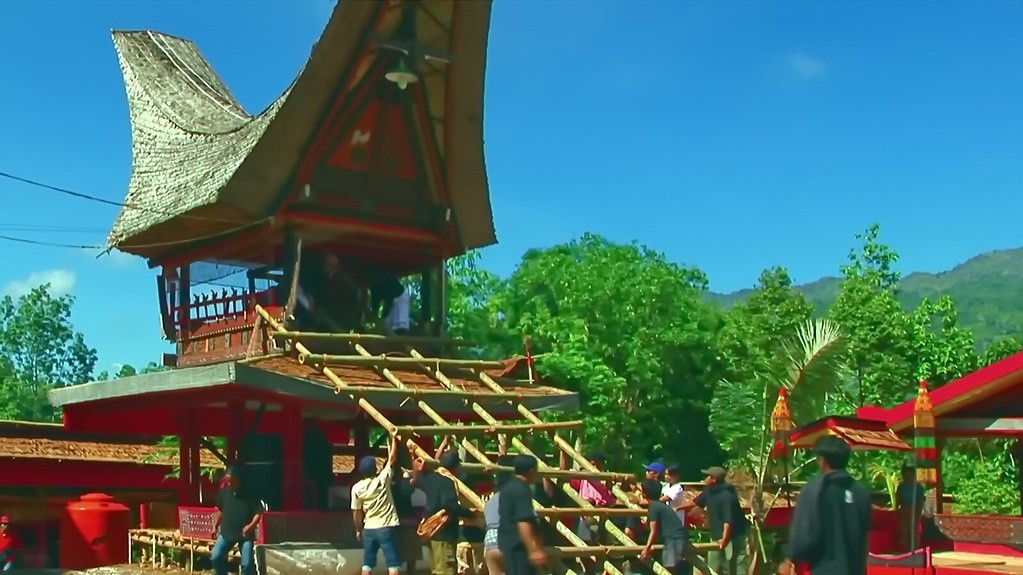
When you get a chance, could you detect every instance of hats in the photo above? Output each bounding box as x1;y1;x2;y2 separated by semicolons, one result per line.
702;467;726;481
441;450;463;468
360;455;377;475
490;471;508;490
642;462;664;472
0;516;10;524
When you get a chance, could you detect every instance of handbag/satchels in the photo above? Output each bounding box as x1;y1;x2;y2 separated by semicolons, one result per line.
416;508;451;542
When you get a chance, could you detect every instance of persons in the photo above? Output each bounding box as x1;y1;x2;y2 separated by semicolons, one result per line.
0;514;25;575
893;467;925;554
209;461;264;575
349;427;744;575
777;437;874;575
